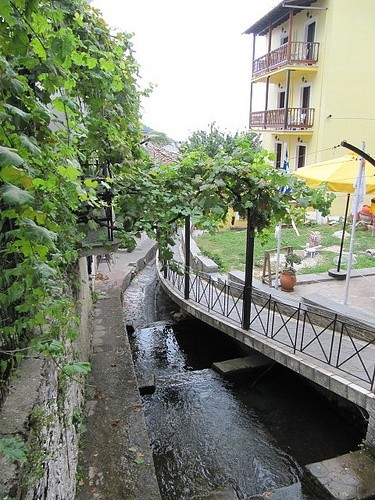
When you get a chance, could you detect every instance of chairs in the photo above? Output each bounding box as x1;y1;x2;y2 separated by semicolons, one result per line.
291;113;307;131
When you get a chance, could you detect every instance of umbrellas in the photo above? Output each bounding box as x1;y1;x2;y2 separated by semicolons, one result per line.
293;150;374;272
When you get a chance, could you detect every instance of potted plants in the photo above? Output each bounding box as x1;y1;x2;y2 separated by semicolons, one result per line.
280;252;304;292
